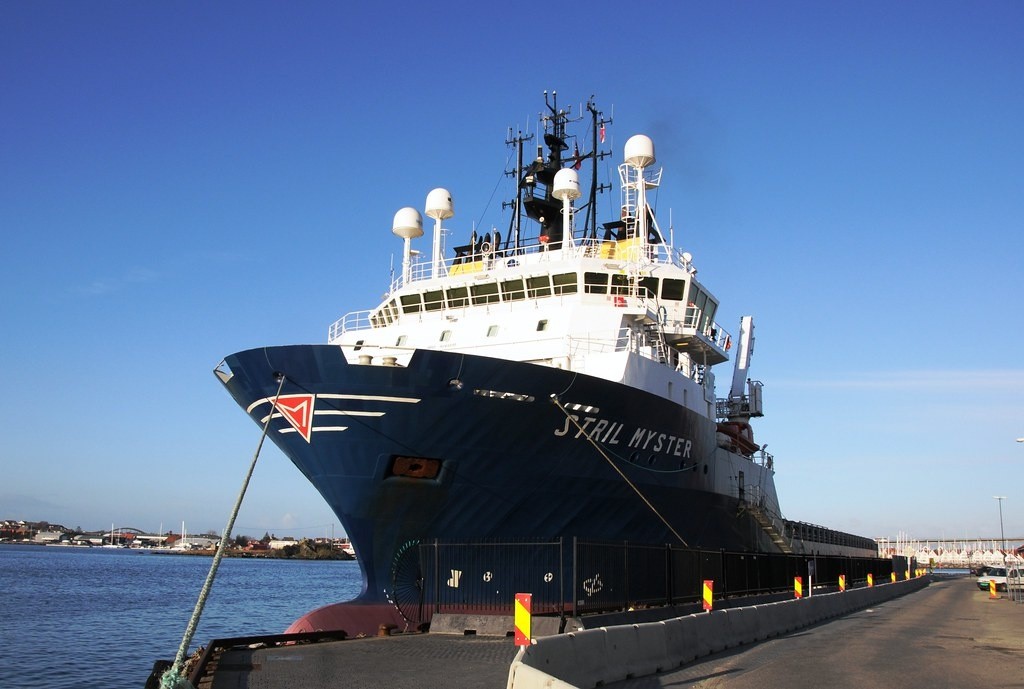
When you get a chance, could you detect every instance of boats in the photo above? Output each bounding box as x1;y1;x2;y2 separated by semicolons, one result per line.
212;90;893;635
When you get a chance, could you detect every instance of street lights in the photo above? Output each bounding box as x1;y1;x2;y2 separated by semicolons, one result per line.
992;496;1007;567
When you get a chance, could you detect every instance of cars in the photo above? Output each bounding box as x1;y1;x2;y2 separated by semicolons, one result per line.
977;564;1010;591
1007;565;1024;591
